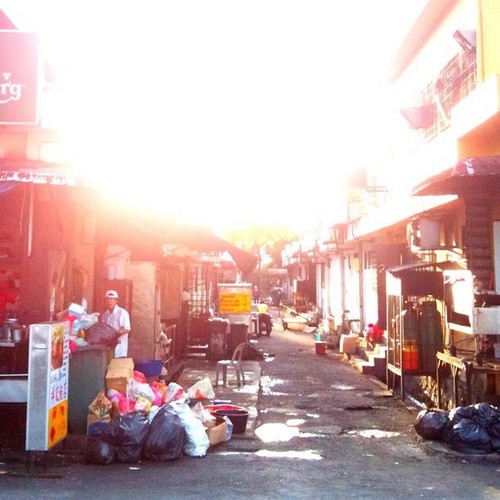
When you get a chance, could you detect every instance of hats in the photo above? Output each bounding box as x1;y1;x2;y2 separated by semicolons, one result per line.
105;290;118;298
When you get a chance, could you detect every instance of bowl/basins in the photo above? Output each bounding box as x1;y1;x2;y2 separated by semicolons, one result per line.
135;360;164;376
204;404;250;433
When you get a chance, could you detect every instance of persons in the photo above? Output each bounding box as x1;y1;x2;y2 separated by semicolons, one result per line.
256;300;268;314
102;290;131;359
253;285;260;303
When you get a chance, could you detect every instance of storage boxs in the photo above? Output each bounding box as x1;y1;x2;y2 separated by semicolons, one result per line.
202;416;226;445
105;358;135;395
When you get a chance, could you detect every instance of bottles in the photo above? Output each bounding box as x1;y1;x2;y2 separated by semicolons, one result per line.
0;318;26;342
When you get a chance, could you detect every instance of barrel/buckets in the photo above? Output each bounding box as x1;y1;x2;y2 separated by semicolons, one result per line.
315;341;327;353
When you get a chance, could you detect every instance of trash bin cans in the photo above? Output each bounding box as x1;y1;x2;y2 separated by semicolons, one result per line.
229;322;250;353
208;318;228;360
67;344;117;436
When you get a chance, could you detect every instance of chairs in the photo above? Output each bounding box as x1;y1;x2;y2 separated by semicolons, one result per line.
215;343;245;387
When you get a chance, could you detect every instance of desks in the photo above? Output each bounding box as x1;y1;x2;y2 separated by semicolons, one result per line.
436;351;500;410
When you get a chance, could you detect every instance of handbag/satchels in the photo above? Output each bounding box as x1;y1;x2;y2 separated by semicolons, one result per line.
128;372;216;412
